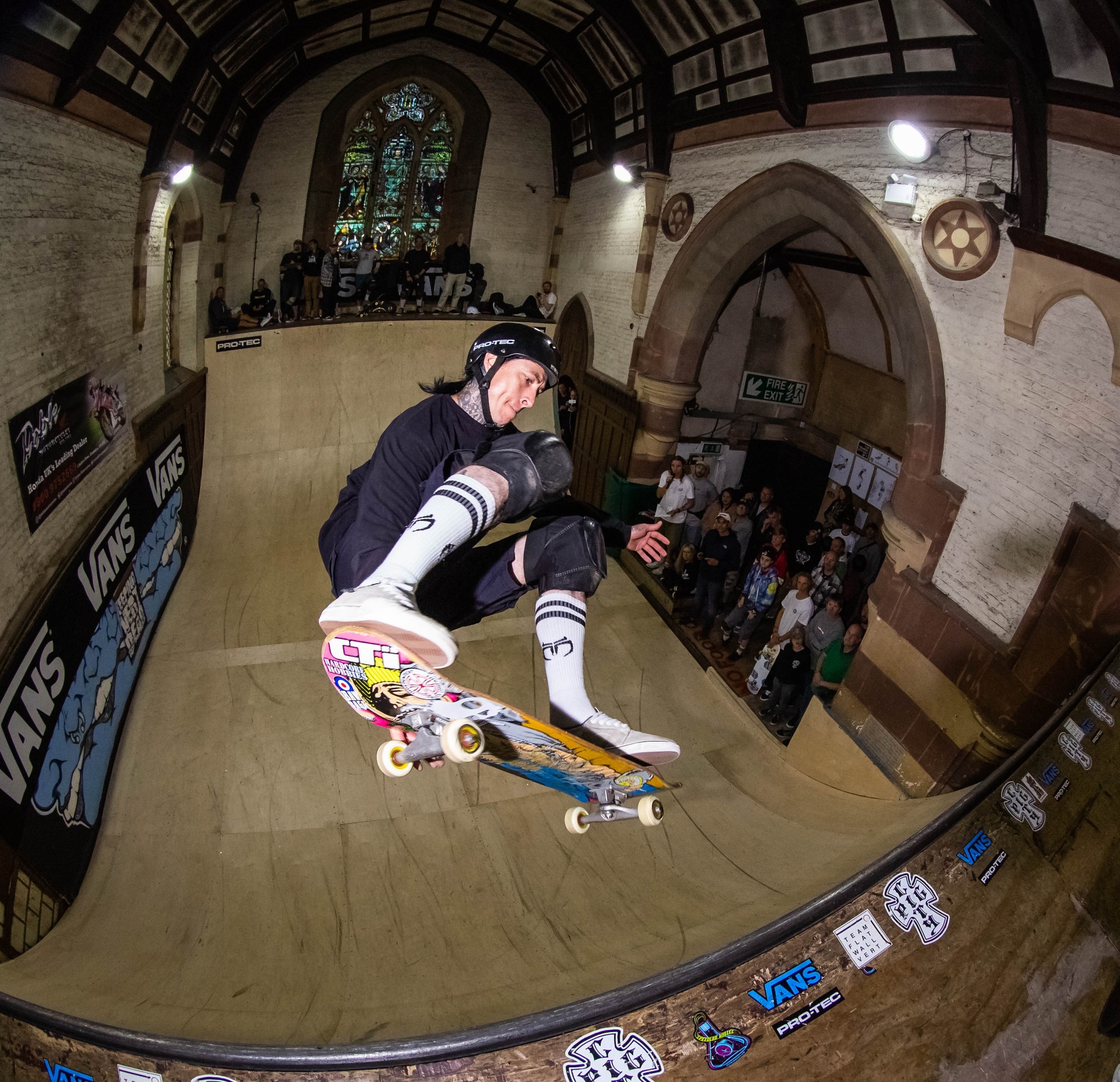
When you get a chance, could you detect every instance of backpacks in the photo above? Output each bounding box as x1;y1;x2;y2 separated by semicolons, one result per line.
488;292;506;313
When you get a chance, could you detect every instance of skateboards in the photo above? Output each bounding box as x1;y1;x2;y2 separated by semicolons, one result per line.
744;640;781;695
319;624;682;836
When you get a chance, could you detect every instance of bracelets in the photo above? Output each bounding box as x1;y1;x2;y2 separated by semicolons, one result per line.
780;636;783;642
406;275;410;278
418;274;420;277
773;631;778;633
752;610;756;614
677;509;680;513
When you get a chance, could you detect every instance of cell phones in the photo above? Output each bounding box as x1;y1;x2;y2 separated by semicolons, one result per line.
699;554;707;558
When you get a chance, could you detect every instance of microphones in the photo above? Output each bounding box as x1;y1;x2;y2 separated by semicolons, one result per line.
666;472;675;489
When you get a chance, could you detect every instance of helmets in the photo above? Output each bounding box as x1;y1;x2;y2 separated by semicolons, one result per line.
695;460;708;467
464;322;562;392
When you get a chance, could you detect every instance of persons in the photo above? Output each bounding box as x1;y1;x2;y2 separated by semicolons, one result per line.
318;322;681;766
241;279;277;327
432;233;471;315
209;286;238;332
646;455;884;747
395;236;430;316
88;377;124;418
493;281;557;319
347;237;381;315
558;383;578;445
279;239;341;323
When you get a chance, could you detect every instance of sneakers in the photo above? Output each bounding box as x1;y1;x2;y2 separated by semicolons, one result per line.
466;306;482;314
318;578;460;669
277;309;285;323
397;308;406;315
564;707;681;768
416;306;425;315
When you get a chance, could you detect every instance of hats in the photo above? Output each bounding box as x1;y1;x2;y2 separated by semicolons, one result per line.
715;513;731;523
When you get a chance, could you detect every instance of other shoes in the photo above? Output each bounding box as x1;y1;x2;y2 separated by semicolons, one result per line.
448;309;460;315
301;314;341;321
512;312;527;317
729;650;743;662
760;689;771;701
492;302;505;315
652;563;672;575
759;708;775;717
770;714;781;726
786;717;799;729
285;318;295;323
775;729;793;738
260;312;273;327
432;309;443;315
721;626;733;643
646;557;666;568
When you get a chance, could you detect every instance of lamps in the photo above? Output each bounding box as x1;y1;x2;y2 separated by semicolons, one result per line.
612;163;636;183
887;120;932;164
163;159;194;183
